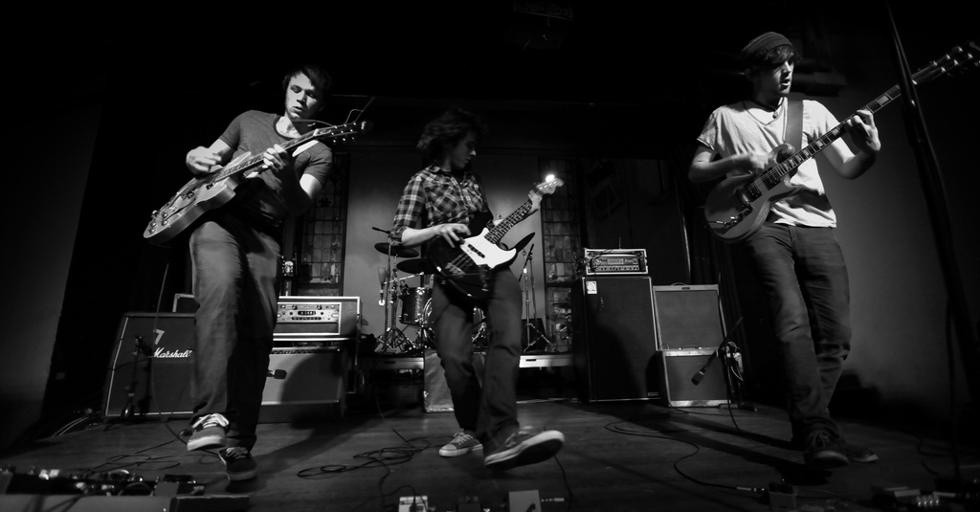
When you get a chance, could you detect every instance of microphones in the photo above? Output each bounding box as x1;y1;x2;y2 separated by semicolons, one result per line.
691;316;749;388
291;116;316;125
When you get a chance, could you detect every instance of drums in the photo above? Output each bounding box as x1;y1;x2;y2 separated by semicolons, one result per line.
469;308;491;346
400;286;431;325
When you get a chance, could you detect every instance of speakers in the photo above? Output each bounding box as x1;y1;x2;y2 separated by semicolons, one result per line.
664;349;735;408
99;311;196;424
652;282;730;351
257;343;362;425
581;273;661;408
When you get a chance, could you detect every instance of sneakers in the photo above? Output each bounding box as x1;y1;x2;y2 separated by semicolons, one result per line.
439;430;484;456
805;429;848;470
187;412;229;451
837;435;880;462
485;428;564;465
218;446;258;480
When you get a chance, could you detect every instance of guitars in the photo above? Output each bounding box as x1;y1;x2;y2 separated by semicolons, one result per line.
704;46;972;243
143;122;367;243
433;177;566;299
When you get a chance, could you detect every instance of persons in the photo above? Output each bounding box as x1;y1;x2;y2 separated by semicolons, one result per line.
185;62;332;480
389;112;565;469
689;30;881;465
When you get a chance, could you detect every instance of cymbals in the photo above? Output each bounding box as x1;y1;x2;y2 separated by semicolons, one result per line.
398;259;435;274
374;243;418;257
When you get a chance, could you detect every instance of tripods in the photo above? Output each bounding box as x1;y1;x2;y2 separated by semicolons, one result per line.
376;256;399;353
522;266;552;353
384;267;414;353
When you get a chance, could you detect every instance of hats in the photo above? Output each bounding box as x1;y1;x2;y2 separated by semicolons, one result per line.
743;31;792;73
